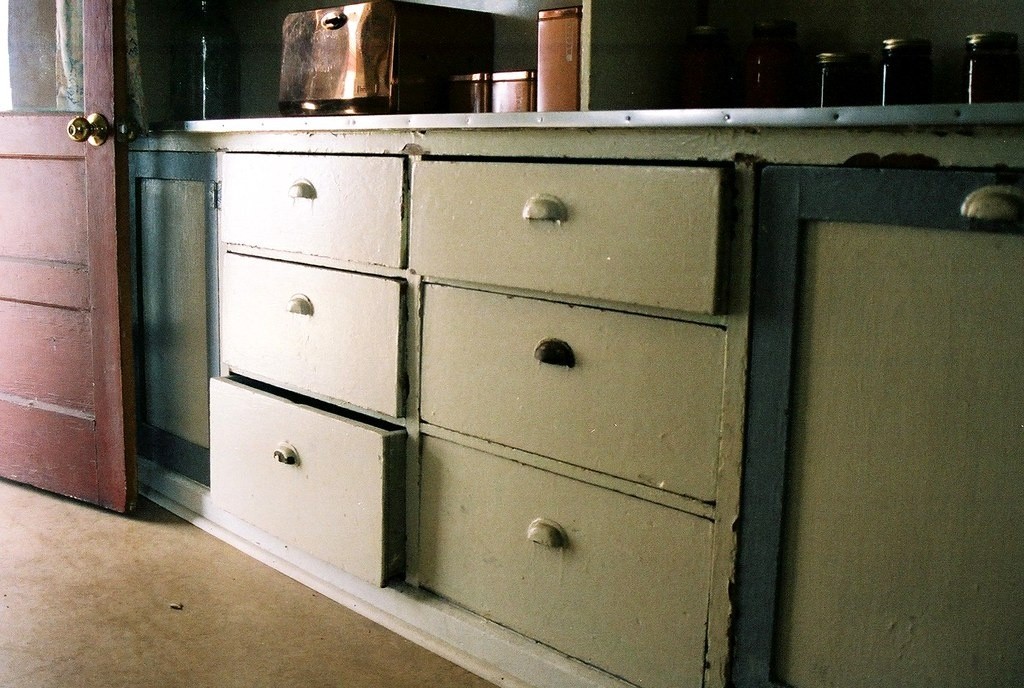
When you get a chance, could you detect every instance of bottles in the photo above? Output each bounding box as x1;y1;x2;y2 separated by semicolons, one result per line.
962;31;1019;103
882;38;933;105
746;19;800;108
812;52;847;107
171;0;241;122
682;25;732;108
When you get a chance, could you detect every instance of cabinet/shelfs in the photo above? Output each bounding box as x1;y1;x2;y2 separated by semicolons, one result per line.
127;119;1024;688
